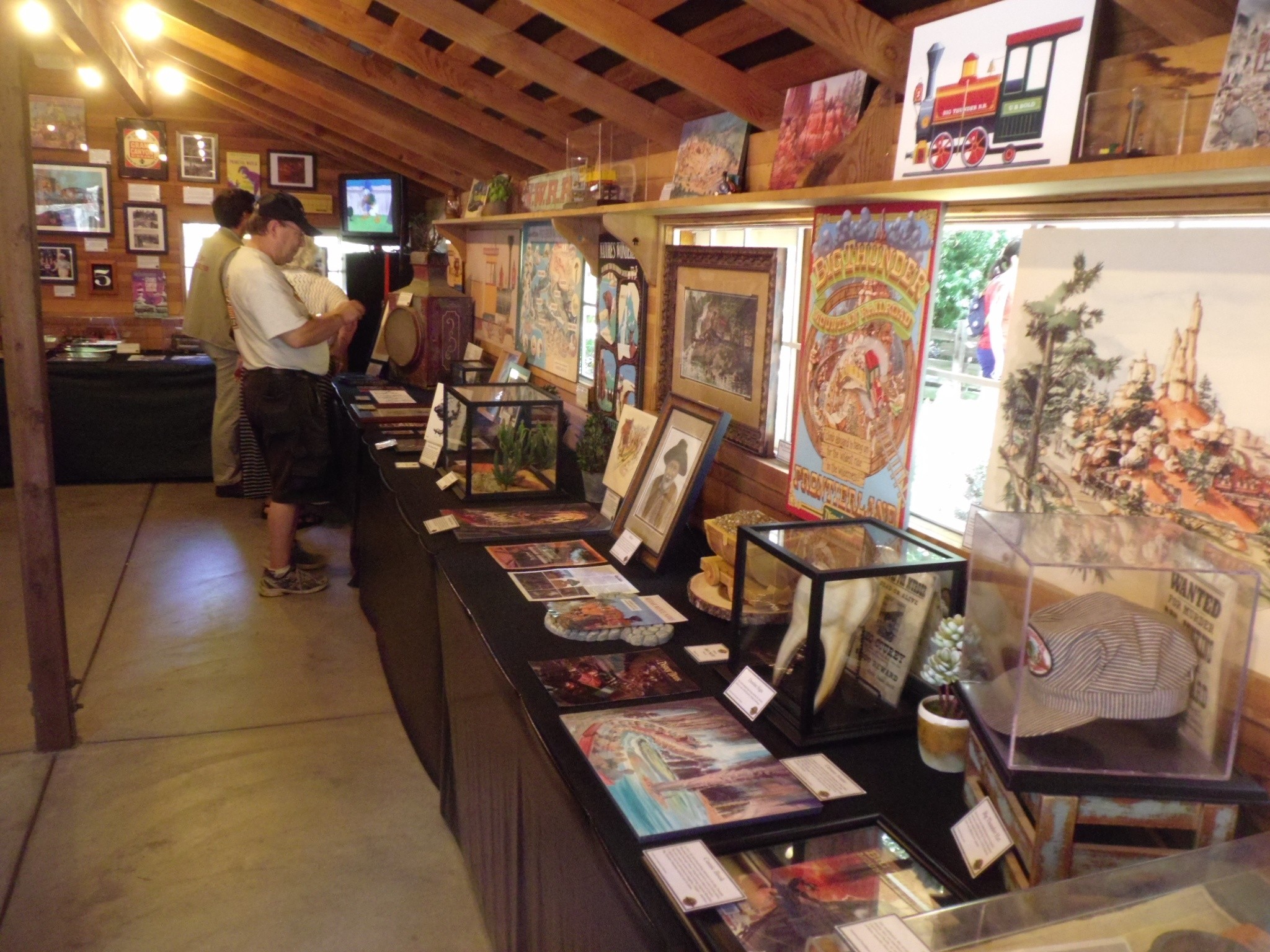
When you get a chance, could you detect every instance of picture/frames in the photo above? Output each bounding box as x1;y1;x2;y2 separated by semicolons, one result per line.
175;130;220;182
656;244;788;458
266;149;317;191
609;391;732;577
476;347;532;431
642;812;978;952
116;117;169;181
31;160;114;237
29;92;88;154
38;242;119;295
123;201;168;255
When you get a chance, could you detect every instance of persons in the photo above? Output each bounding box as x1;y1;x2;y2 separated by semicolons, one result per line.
181;189;258;498
220;190;365;595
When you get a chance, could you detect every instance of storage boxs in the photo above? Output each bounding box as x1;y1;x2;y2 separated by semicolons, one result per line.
564;121;648;208
960;513;1260;774
437;382;562;503
450;359;494;385
729;517;967;750
1079;85;1189;158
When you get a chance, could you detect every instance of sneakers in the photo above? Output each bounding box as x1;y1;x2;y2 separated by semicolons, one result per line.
290;540;327;570
258;564;328;597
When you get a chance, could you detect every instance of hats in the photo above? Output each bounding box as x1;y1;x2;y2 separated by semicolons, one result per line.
252;191;323;237
967;590;1198;739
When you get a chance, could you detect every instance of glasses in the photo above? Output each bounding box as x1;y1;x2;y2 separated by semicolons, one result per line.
284;223;304;241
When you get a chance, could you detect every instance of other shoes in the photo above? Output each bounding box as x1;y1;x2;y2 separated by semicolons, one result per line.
261;501;324;526
215;481;244;498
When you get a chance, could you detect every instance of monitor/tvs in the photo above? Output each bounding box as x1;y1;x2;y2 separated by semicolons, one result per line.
339;171;406;240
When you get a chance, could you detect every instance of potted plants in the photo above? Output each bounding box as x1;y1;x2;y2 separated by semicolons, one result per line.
918;613;969;775
484;175;512;215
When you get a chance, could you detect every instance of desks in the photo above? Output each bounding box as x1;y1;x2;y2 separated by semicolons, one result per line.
1;344;214;483
330;371;973;951
